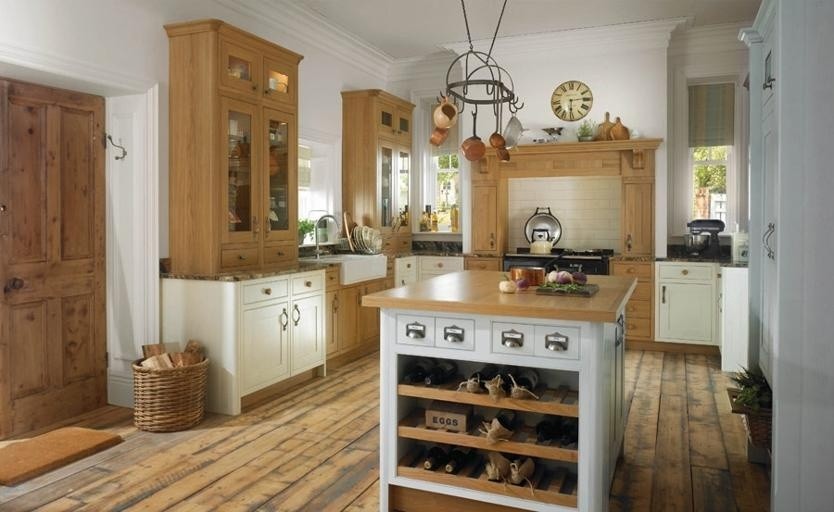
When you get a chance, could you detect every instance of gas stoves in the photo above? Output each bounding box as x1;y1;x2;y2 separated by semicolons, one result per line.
505;251;603;261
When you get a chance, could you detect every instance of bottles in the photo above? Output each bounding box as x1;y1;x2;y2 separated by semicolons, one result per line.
270;128;283;141
450;204;459;232
270;195;286;210
423;444;448;470
400;358;459;386
535;413;579;447
228;170;238;185
488;406;517;440
420;211;428;231
488;233;496;250
624;233;633;256
232;135;249;161
468;364;540;399
432;212;438;231
445;447;474;474
404;205;408;225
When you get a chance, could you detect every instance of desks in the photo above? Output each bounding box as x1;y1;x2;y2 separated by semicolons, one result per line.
362;270;640;512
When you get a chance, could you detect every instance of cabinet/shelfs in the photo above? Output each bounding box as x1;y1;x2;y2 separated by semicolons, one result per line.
654;258;720;348
464;139;665;351
341;89;416;253
395;258;417;287
719;266;750;373
739;0;834;394
419;257;464;282
325;261;395;369
161;269;326;415
163;18;300;277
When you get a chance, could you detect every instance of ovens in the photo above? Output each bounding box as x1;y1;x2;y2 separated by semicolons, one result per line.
503;258;608;275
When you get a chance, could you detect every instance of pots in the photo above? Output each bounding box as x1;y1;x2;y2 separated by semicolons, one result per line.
429;128;448;147
461;113;486;161
509;251;566;286
524;206;562;247
502;111;523;150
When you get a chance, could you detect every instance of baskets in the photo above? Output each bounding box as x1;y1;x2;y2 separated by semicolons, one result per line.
742;404;772;449
132;358;207;432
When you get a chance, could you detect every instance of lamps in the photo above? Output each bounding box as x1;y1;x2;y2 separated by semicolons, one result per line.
429;0;525;163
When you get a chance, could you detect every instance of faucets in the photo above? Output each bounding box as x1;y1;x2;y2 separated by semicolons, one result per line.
313;214;340;259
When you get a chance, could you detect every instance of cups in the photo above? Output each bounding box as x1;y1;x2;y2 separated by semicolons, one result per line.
232;68;241;78
383;178;389;187
268;76;287;92
229;119;240;136
382;155;388;164
382;198;388;208
339;238;349;250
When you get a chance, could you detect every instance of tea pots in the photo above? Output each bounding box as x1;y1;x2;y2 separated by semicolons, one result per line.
529;228;555;255
433;95;459;131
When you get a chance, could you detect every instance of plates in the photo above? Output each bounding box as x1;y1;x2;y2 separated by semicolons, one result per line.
338;249;351;254
350;224;383;256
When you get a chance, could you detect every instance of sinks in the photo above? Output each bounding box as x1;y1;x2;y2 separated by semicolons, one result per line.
299;253;389;286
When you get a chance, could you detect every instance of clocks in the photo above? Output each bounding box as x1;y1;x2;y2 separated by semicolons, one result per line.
551;81;593;121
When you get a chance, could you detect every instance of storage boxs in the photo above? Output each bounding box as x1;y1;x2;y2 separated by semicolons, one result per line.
727;388;772;465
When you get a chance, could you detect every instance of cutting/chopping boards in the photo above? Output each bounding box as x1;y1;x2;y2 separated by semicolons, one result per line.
598;111;615;141
535;283;600;298
609;116;629;140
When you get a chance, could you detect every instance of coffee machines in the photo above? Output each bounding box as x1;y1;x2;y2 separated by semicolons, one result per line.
684;219;727;260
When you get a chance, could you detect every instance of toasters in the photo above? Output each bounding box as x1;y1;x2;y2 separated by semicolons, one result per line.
730;231;749;267
